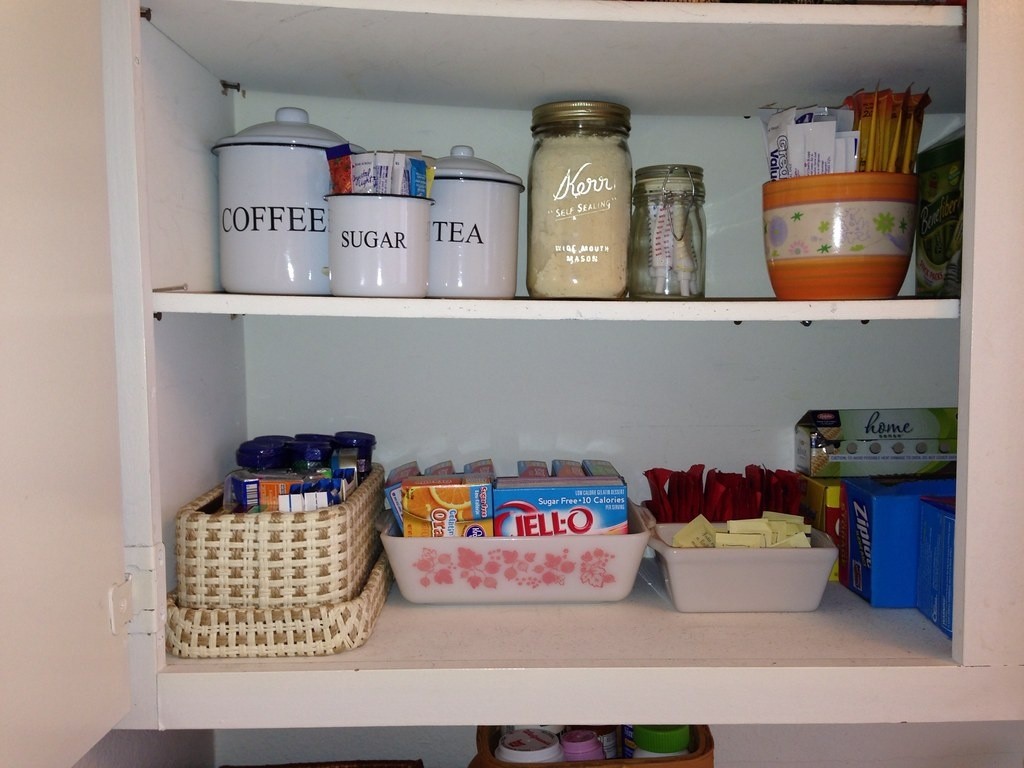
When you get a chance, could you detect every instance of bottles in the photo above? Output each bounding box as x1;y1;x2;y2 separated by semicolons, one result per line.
526;100;634;300
495;725;692;762
627;164;707;299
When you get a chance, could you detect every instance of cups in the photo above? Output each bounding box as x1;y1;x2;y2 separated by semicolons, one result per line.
211;106;366;297
428;144;524;299
323;193;435;298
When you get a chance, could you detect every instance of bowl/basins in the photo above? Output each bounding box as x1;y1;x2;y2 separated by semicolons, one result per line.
646;523;839;614
761;171;921;300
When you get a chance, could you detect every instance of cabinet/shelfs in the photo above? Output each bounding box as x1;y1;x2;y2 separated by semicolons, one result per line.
95;0;1024;730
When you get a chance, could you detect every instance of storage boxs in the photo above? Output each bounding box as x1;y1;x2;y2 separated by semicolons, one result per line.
384;459;628;537
350;150;437;196
231;467;358;513
796;407;958;477
648;522;839;612
620;725;637;759
915;137;966;298
800;471;956;639
376;498;656;604
